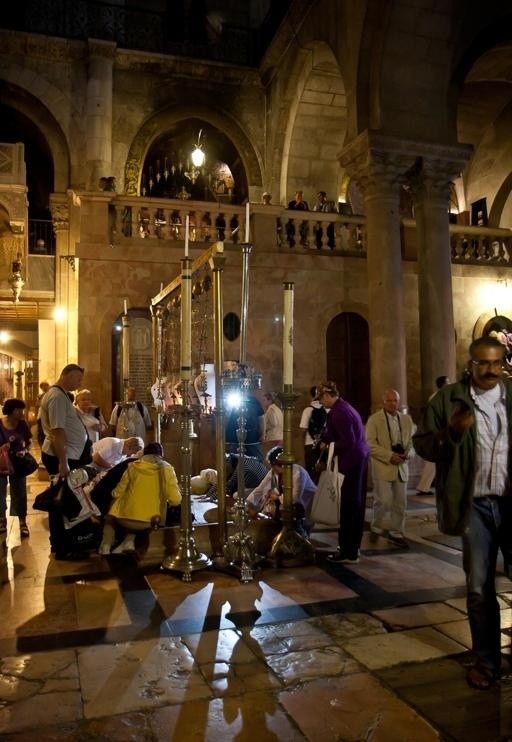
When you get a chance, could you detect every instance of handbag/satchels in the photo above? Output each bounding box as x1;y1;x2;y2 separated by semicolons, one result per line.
10;453;39;480
314;444;334;479
50;473;93;537
77;440;92;466
310;441;345;526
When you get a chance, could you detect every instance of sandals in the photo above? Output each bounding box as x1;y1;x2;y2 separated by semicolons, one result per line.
20;524;30;537
1;518;7;533
463;655;497;690
416;491;433;495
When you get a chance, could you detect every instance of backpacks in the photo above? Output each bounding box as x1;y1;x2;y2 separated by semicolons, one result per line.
307;405;327;440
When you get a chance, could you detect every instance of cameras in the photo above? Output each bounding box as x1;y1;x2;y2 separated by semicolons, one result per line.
393;444;403;453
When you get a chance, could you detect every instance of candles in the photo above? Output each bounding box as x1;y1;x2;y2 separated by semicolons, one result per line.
160;282;164;292
183;215;191;257
124;298;128;316
244;201;251;244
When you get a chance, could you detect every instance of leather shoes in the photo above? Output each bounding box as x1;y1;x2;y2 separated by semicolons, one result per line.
326;551;360;565
336;544;360;556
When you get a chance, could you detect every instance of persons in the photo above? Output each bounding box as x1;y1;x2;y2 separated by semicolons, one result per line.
261;191;273;205
98;175;130;222
288;190;310;247
312;190;337;250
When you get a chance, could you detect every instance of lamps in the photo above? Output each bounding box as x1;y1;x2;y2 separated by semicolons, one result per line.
186;126;209;169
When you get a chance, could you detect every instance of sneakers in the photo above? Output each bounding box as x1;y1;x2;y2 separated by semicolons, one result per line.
99;543;111;556
388;533;408;547
369;531;380;544
112;541;136;554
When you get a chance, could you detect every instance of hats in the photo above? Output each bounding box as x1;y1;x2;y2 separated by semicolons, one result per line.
312;381;337;401
